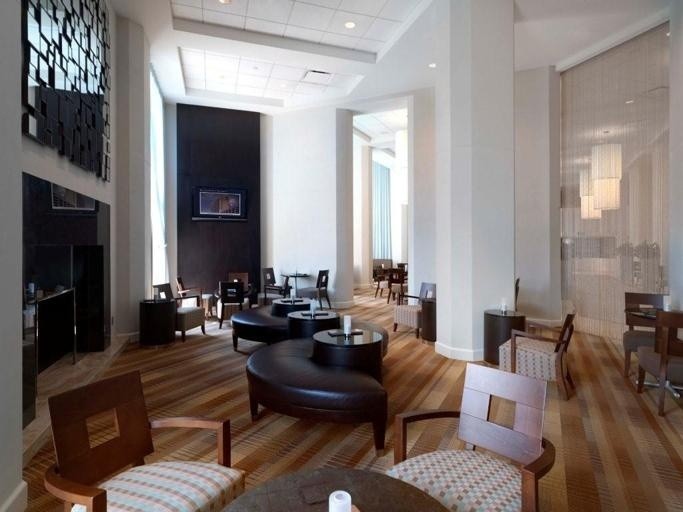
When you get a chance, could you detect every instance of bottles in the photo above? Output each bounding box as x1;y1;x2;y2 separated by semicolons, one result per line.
310;300;316;316
343;315;351;337
290;289;295;303
502;303;507;315
28;283;35;301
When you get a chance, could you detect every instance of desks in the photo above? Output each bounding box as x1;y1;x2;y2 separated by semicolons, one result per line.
217;467;449;511
483;310;526;365
421;298;436;343
288;310;340;337
312;328;384;384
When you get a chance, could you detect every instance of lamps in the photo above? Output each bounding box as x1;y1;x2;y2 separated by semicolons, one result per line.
592;130;622;211
577;158;603;220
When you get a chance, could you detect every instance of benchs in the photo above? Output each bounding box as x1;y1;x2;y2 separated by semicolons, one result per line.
341;321;389;359
231;336;387;450
230;306;289;351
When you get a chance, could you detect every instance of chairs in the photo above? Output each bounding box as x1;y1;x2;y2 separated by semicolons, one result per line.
384;362;556;511
393;282;437;339
622;292;683;417
152;267;332;343
372;258;408;305
43;370;246;512
498;309;578;398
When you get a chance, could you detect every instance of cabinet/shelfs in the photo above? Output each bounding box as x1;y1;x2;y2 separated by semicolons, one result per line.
139;299;177;349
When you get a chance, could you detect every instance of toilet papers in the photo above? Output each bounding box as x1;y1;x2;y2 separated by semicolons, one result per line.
28;282;35;294
328;490;352;512
290;289;295;301
310;298;317;314
500;297;507;312
343;315;352;335
663;295;672;312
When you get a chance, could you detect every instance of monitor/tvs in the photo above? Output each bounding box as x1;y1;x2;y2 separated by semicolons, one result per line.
192;185;249;224
